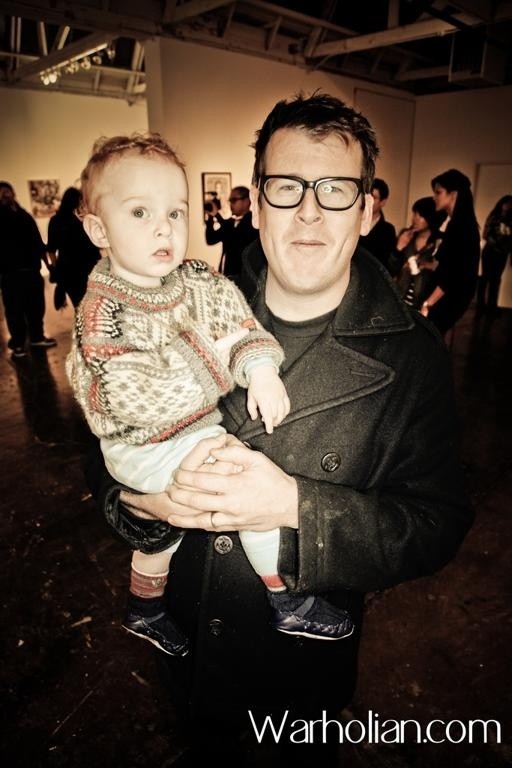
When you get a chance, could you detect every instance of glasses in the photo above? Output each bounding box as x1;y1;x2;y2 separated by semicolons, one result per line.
258;174;363;211
229;198;245;202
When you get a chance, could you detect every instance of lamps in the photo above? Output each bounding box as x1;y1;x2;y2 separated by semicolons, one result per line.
39;42;116;85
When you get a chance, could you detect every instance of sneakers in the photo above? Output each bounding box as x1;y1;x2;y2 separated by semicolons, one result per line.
266;589;355;641
29;338;56;347
12;347;28;357
121;595;190;657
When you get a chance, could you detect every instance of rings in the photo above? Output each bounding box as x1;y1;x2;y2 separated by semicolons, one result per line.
210;512;216;529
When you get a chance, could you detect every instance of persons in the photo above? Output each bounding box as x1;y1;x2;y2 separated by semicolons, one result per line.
0;182;98;358
157;91;480;768
64;132;355;658
370;169;512;339
205;186;257;274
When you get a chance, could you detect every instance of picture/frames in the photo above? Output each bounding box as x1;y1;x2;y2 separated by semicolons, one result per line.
202;173;232;223
